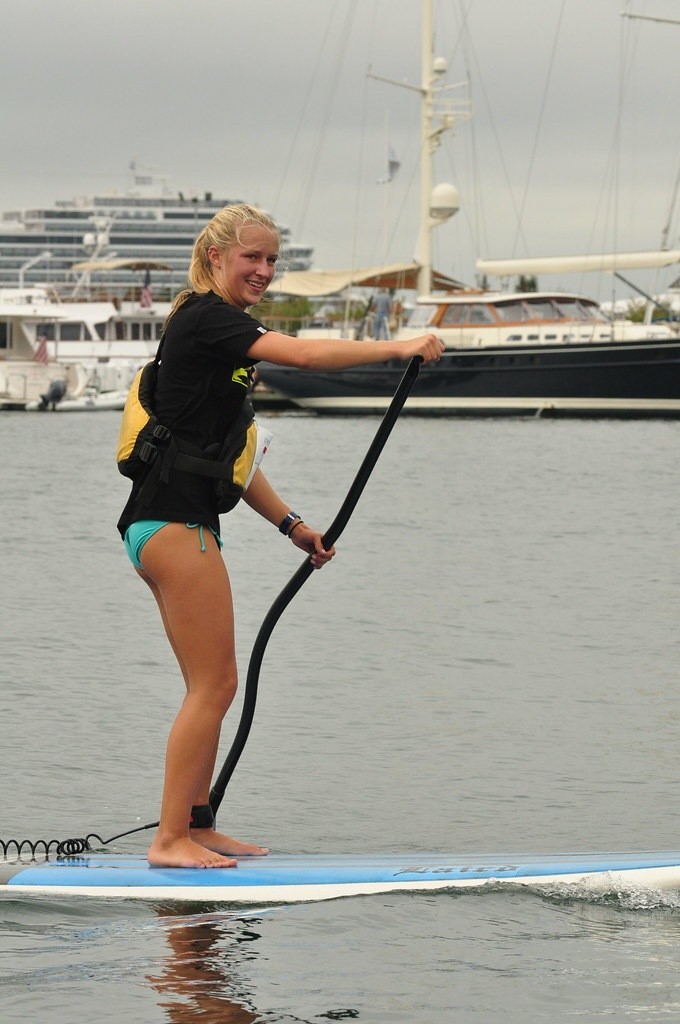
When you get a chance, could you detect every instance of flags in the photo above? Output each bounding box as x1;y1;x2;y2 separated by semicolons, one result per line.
32;335;49;365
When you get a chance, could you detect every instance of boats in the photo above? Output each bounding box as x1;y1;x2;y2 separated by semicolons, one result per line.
0;846;680;909
1;166;313;427
251;1;679;416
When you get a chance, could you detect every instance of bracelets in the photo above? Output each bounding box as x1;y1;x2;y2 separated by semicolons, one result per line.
279;512;304;538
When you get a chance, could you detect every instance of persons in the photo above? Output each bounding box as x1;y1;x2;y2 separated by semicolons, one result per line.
113;203;446;871
